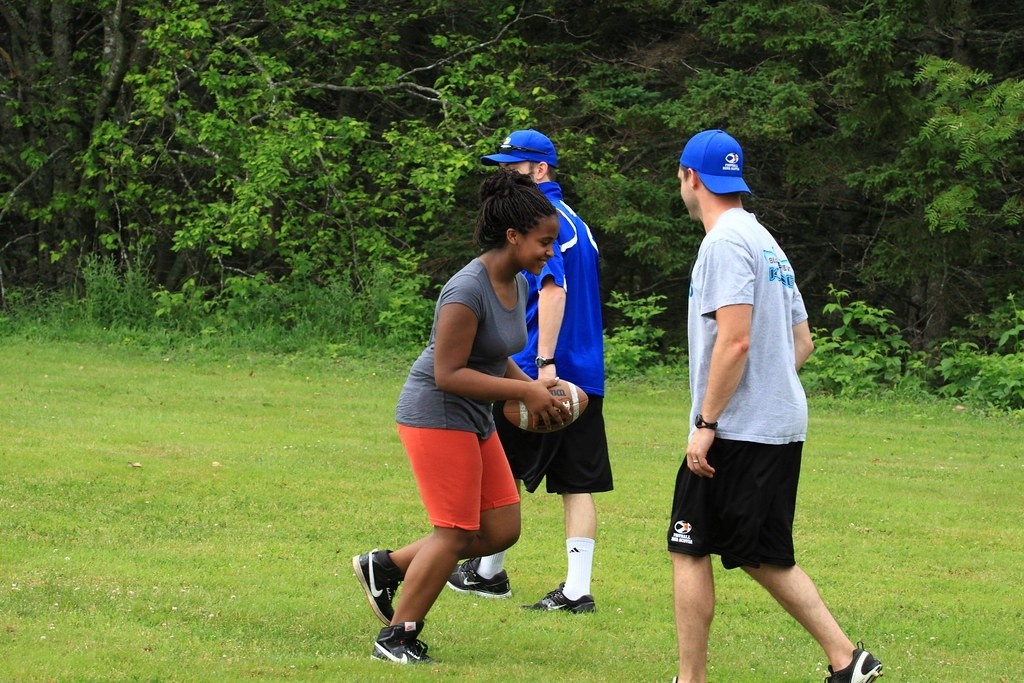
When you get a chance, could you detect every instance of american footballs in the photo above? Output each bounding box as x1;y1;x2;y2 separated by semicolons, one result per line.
502;378;589;434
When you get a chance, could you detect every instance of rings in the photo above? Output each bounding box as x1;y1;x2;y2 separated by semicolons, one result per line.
692;459;700;466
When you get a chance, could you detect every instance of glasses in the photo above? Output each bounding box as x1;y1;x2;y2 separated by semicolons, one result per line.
499;144;548;156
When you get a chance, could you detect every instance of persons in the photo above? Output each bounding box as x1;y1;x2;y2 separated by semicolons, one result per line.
668;130;887;683
350;171;574;663
440;128;615;618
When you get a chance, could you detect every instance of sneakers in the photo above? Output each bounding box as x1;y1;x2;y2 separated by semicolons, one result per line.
521;581;596;613
446;556;512;599
829;648;882;683
351;549;404;627
369;621;437;666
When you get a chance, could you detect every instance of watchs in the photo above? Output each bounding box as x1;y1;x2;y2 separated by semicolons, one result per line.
534;355;556;368
694;414;719;432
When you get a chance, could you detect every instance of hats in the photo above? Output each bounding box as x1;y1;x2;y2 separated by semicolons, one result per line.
679;130;753;196
479;129;558;168
